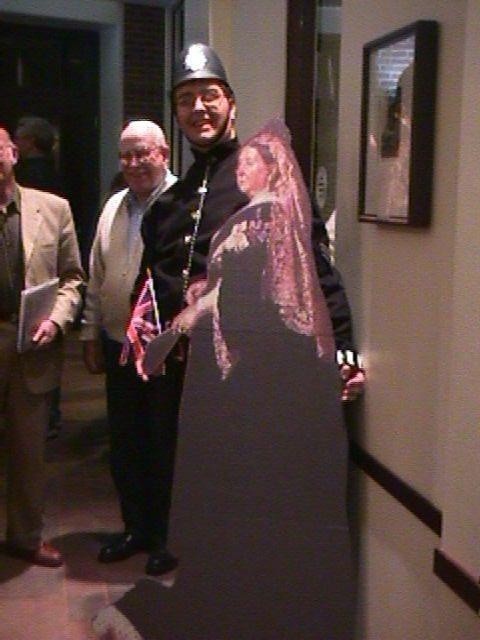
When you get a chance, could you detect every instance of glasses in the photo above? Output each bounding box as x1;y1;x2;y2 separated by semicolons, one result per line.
173;89;228;106
119;145;162;166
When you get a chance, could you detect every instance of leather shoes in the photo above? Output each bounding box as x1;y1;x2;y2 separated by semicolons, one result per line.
145;541;179;575
4;540;63;565
99;532;147;563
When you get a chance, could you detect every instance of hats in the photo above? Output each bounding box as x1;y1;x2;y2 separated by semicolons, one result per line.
173;44;234;95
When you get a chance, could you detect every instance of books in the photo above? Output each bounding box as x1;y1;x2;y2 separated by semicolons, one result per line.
16;275;60;354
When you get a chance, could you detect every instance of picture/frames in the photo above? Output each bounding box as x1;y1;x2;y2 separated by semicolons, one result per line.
357;19;440;229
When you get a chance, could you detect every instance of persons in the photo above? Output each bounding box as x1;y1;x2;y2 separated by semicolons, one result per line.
0;127;86;569
81;119;183;580
95;118;364;639
131;40;365;541
9;117;76;442
